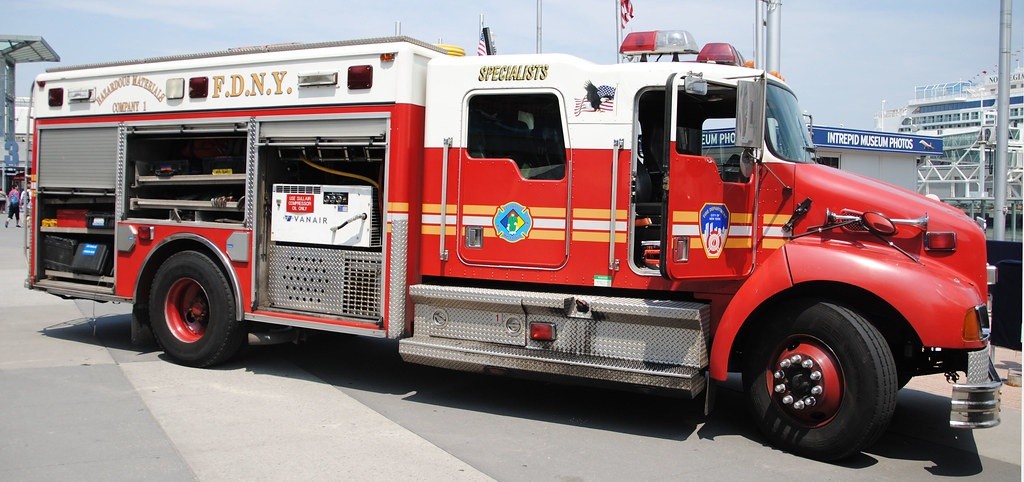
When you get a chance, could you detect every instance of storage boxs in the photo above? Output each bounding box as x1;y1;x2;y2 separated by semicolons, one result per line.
42;234;79;264
71;243;111;276
54;210;88;227
201;156;246;174
145;159;193;175
44;258;71;272
85;211;114;230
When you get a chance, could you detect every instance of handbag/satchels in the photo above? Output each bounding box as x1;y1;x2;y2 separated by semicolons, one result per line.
10;194;19;209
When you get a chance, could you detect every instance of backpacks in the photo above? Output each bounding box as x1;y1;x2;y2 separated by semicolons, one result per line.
21;191;30;205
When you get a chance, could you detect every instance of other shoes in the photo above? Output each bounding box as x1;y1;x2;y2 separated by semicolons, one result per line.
5;221;8;228
16;225;21;227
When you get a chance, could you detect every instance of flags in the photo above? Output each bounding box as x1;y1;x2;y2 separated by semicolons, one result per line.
477;20;497;56
621;0;634;30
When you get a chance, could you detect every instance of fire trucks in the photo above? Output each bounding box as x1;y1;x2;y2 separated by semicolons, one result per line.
19;27;998;465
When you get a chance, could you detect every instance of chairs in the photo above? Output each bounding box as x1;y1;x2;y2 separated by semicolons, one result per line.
487;118;538;169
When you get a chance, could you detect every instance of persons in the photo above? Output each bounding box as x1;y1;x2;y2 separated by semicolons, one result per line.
0;182;31;228
